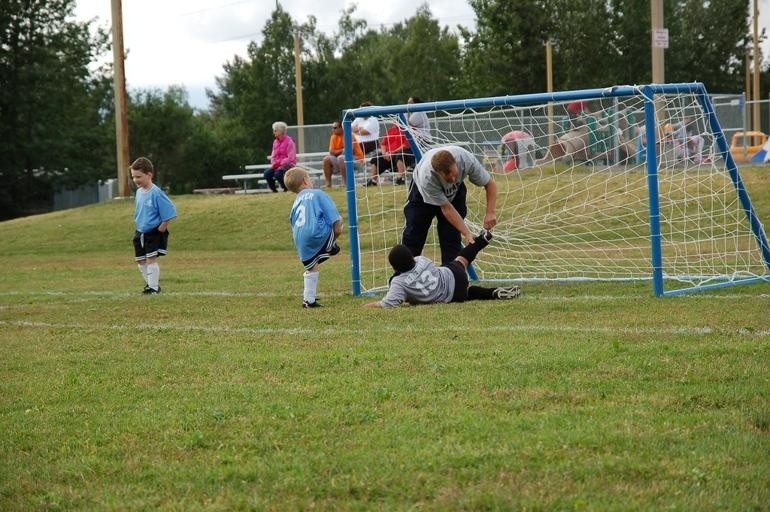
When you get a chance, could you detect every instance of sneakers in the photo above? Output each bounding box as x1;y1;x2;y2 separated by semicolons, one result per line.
492;285;522;299
362;179;377;187
482;229;492;242
395;180;405;186
301;300;321;307
142;284;161;294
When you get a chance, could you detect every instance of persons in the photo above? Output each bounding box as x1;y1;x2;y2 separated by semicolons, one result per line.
388;146;498;289
285;166;344;310
407;96;432;147
364;229;522;311
362;114;416;187
128;156;178;294
673;111;705;162
263;122;298;193
323;119;366;191
350;101;380;158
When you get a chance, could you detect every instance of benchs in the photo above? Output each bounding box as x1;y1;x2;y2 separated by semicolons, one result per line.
222;141;471;194
